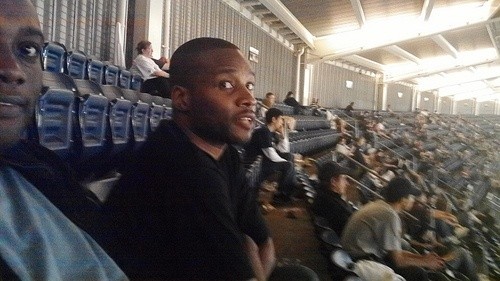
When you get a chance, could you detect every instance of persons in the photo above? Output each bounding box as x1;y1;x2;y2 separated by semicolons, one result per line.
312;160;359;238
335;102;500;281
129;40;173;99
257;91;308;134
310;98;326;116
247;107;296;203
342;176;446;281
103;37;320;281
0;0;130;281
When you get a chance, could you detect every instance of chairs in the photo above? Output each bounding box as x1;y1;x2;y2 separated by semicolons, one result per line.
23;42;500;280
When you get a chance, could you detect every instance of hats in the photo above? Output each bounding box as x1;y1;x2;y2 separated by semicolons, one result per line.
390;176;421;196
317;160;351;180
